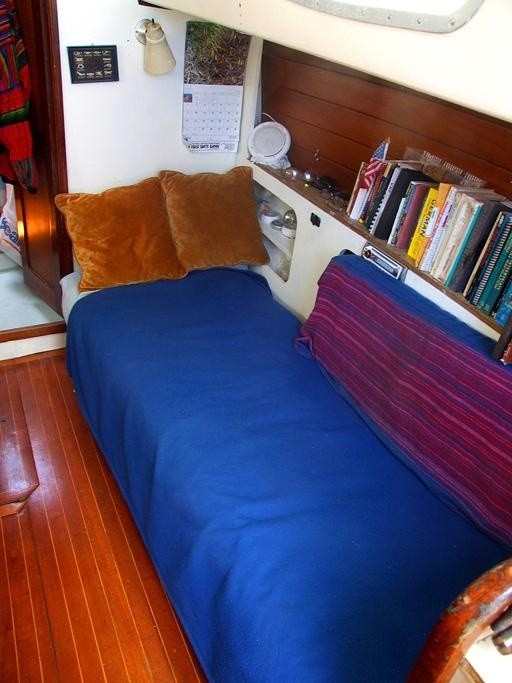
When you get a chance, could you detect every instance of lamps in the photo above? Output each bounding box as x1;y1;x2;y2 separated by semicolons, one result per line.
136;18;177;78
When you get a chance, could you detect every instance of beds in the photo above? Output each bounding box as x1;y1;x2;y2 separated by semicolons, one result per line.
60;265;512;683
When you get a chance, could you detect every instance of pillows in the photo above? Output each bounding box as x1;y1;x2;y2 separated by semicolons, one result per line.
159;164;273;268
51;177;188;293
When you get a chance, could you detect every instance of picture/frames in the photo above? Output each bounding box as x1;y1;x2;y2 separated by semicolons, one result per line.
68;44;120;83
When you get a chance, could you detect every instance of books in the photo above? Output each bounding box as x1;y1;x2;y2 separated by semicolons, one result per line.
346;160;512;367
